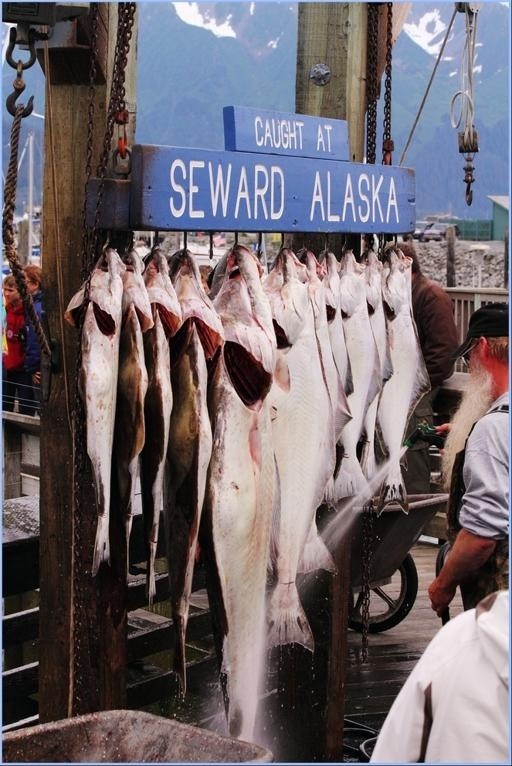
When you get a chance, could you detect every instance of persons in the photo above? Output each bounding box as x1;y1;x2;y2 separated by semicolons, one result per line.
21;266;44;414
372;589;509;764
3;274;36;410
428;300;511;616
381;243;458;409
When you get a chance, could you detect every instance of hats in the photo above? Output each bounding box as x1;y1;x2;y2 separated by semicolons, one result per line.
450;303;509;360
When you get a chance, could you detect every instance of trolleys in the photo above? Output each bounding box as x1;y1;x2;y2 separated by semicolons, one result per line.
342;485;456;633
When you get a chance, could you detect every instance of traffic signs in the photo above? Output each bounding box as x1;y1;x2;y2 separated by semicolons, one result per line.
127;144;421;233
219;106;353;161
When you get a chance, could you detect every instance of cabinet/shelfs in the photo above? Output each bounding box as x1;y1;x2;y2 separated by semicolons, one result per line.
1;495;217;732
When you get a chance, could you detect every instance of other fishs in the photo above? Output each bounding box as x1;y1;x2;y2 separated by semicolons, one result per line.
64;245;433;742
